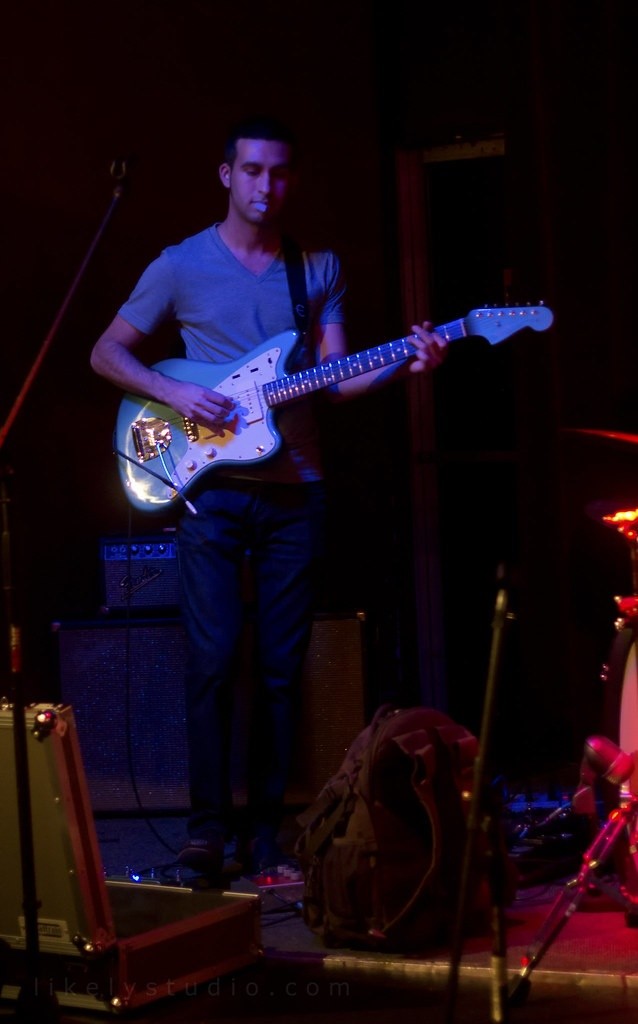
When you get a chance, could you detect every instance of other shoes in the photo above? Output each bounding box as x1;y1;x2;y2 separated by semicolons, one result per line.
176;837;226;878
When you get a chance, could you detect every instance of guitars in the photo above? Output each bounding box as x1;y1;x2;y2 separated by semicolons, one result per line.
115;303;554;513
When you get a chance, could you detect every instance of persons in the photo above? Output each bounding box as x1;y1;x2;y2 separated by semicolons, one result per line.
90;120;450;899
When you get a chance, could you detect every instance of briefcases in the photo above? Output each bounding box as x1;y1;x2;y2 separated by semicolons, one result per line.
0;695;263;1014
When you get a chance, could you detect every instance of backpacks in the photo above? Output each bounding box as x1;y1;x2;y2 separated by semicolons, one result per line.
294;707;497;947
